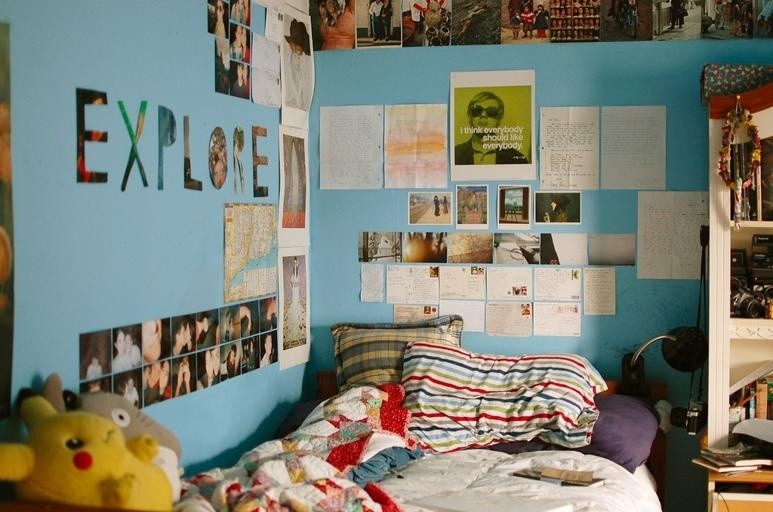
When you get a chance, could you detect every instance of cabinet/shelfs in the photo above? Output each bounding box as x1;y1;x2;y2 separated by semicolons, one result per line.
709;82;773;512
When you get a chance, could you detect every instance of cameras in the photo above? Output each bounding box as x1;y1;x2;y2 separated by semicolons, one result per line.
670;401;707;435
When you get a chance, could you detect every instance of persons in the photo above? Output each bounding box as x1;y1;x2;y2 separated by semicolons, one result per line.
432;195;448;218
79;297;278;410
286;255;307;342
311;1;754;50
542;195;570;223
455;91;527;164
207;1;252;100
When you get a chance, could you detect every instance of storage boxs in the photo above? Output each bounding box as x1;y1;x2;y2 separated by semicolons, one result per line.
701;63;772;105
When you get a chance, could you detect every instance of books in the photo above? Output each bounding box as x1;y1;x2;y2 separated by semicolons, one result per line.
691;378;773;475
508;464;608;488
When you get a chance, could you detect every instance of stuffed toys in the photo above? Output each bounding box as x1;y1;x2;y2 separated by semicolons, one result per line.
0;374;185;512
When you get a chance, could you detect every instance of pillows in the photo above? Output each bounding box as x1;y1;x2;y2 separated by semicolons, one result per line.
402;339;608;454
330;315;463;394
488;393;661;474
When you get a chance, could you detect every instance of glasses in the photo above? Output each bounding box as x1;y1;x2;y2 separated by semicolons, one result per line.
468;105;499;119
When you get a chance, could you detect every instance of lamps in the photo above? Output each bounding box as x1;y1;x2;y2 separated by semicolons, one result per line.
621;325;708;399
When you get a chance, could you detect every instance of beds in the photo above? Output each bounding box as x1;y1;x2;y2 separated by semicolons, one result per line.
169;370;663;511
